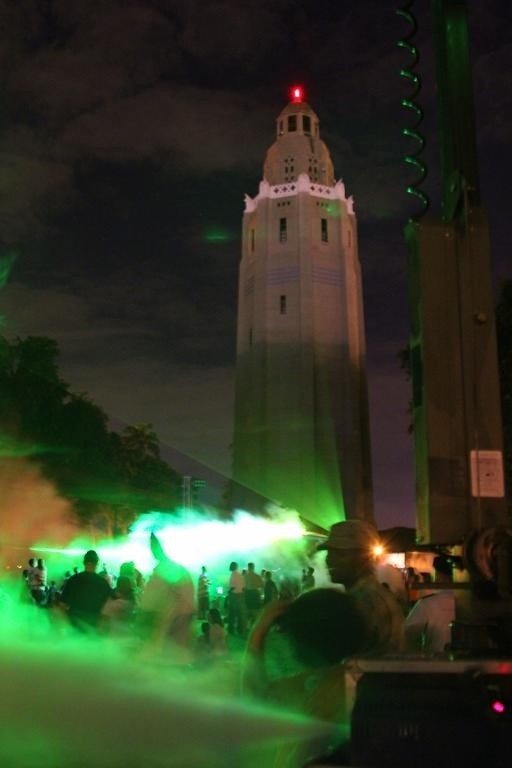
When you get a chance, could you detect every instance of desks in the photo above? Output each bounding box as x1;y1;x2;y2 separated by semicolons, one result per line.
351;652;512;768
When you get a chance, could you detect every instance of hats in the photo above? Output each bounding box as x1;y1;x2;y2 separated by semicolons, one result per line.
310;517;386;554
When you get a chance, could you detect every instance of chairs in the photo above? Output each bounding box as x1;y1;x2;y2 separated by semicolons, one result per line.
405;591;456;653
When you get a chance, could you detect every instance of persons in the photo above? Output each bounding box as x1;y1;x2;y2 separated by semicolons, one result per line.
16;514;510;729
